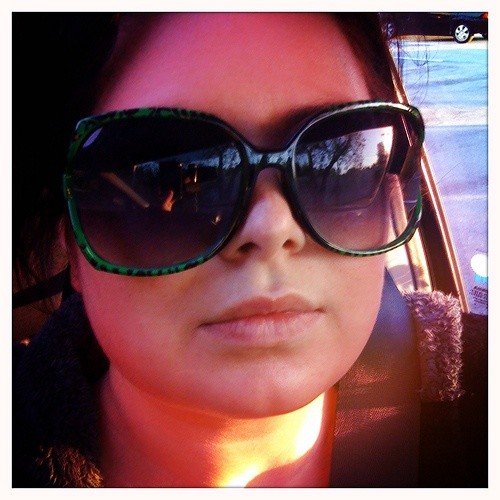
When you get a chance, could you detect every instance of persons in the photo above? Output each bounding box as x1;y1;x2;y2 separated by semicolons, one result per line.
15;10;490;488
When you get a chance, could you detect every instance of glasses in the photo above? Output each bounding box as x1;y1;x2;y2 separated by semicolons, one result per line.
60;99;427;276
